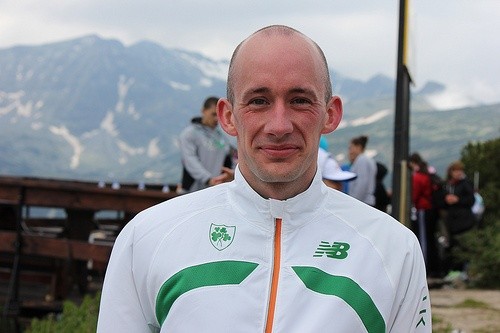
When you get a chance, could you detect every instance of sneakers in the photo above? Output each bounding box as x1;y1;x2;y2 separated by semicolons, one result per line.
444;271;466;281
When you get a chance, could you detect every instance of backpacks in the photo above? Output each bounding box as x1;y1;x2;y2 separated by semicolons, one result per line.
472;193;484;213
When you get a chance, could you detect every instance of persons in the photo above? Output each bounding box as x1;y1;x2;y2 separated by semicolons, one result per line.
178;96;477;278
97;25;433;333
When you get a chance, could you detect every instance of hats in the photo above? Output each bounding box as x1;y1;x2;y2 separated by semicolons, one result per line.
322;156;358;181
